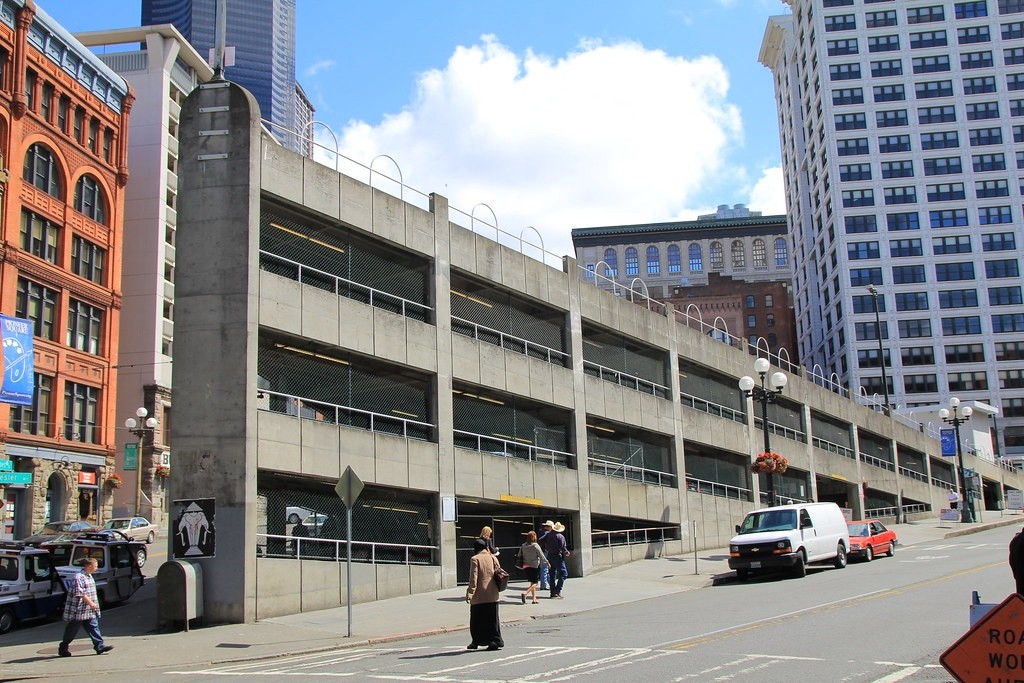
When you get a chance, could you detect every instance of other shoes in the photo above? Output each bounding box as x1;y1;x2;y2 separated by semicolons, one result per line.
58;649;71;656
97;645;113;655
540;587;550;590
487;646;499;650
467;644;478;649
551;594;561;599
554;591;564;598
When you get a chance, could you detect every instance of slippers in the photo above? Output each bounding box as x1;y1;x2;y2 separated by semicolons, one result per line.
532;601;539;604
522;593;526;603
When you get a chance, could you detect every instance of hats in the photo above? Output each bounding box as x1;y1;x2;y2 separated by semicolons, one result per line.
475;539;486;550
552;521;565;532
542;520;554;526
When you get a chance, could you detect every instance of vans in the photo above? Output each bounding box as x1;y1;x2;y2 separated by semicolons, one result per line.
727;501;851;583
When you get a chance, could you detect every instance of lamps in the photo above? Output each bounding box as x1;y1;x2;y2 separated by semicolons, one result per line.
52;461;73;470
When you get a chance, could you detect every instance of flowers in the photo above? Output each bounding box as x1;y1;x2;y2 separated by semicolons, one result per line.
107;473;123;489
155;465;170;479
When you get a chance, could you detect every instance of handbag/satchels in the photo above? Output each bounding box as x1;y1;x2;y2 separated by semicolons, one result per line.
494;568;509;591
487;537;500;556
514;548;523;572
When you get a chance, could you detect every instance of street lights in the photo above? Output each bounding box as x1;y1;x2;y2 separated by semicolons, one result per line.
939;397;973;523
866;283;891;418
125;407;157;518
739;357;788;508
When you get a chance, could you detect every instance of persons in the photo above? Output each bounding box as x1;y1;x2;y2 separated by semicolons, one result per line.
517;531;551;604
537;520;555;590
787;500;793;505
59;557;114;657
947;489;959;509
480;526;499;554
464;539;504;650
807;499;814;503
291;518;309;555
539;522;570;599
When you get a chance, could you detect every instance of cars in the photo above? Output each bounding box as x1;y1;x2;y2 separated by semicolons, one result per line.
24;520;95;550
102;516;158;544
301;514;328;536
39;529;148;574
285;504;314;524
847;520;896;562
